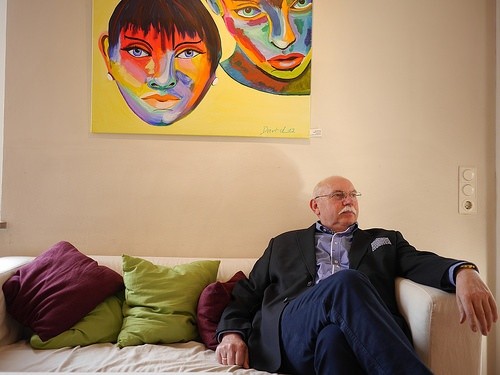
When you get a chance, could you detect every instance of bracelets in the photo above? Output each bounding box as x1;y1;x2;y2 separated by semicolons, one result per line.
454;264;478;282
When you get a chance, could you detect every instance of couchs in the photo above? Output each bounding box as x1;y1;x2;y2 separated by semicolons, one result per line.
0;255;482;375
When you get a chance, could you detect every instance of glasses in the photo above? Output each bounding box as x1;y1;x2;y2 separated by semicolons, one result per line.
315;190;362;201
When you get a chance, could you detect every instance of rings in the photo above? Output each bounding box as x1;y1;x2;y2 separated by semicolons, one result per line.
222;358;226;360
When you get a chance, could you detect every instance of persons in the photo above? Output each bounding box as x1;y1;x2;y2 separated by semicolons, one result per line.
215;176;498;375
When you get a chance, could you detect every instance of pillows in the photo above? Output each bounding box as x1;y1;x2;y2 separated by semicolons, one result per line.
2;241;248;351
